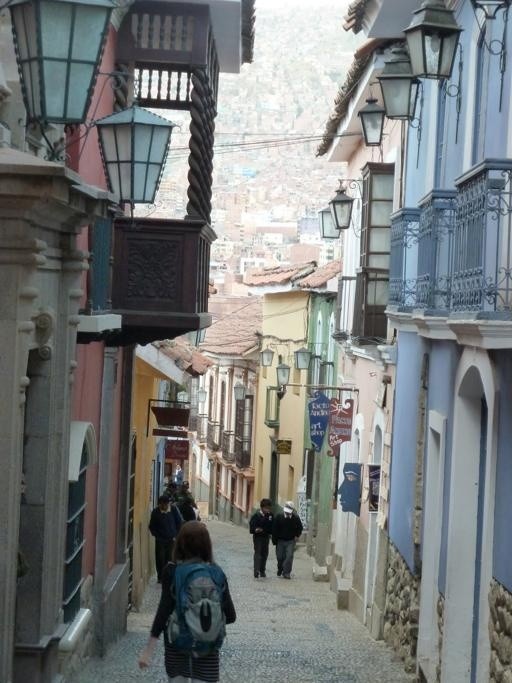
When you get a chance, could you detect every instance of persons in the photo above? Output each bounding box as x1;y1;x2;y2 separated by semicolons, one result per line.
149;496;184;584
249;499;275;577
272;500;302;579
138;519;236;683
164;464;201;522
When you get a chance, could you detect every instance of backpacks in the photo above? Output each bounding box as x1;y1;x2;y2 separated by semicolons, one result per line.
164;558;226;659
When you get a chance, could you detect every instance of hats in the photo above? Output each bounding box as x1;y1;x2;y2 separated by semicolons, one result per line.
283;501;294;513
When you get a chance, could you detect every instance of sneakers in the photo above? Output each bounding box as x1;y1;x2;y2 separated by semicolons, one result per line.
254;570;266;578
277;568;290;578
